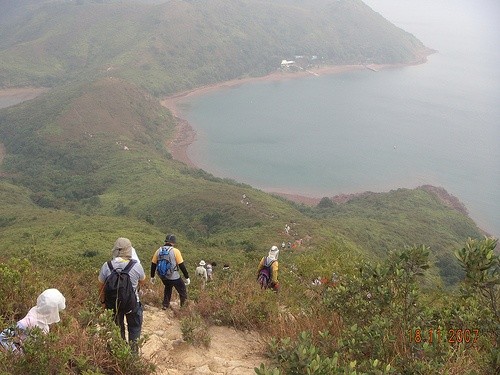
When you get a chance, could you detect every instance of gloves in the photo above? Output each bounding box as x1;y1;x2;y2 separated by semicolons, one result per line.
184;278;190;285
150;277;155;285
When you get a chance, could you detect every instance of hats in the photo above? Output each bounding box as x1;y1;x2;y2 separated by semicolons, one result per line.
164;234;177;243
269;246;279;261
199;261;205;265
33;289;66;324
110;238;132;258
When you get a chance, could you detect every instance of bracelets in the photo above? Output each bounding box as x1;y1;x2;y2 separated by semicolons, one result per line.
99;300;106;304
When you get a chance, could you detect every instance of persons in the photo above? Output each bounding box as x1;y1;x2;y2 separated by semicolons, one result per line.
281;239;302;248
98;237;146;356
150;235;190;310
257;246;279;293
0;288;66;354
195;260;230;291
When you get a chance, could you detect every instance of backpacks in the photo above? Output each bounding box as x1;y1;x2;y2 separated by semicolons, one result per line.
156;247;179;278
103;260;138;315
256;256;277;290
